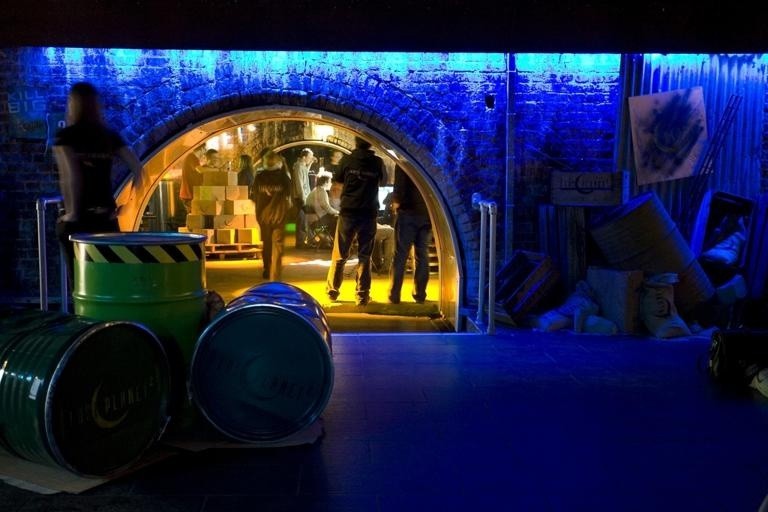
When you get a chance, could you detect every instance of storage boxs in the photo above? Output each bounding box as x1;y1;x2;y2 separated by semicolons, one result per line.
177;170;261;244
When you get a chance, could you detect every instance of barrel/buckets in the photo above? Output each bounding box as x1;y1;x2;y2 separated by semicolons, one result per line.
189;282;335;444
69;233;209;443
0;309;172;481
584;190;718;316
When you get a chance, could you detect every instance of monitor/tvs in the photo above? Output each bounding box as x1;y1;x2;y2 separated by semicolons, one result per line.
377;184;394;210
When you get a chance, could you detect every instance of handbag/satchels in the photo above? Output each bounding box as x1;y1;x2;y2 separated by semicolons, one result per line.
260;192;285;225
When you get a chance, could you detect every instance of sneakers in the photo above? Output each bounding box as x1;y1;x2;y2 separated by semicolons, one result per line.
263;270;269;279
329;294;337;302
296;243;310;248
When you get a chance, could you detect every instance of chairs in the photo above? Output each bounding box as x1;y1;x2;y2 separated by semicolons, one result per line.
301;204;334;253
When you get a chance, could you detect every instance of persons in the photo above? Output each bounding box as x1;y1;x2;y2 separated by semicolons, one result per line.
52;82;149;295
372;223;395;274
388;162;433;304
325;136;389;306
178;143;345;283
